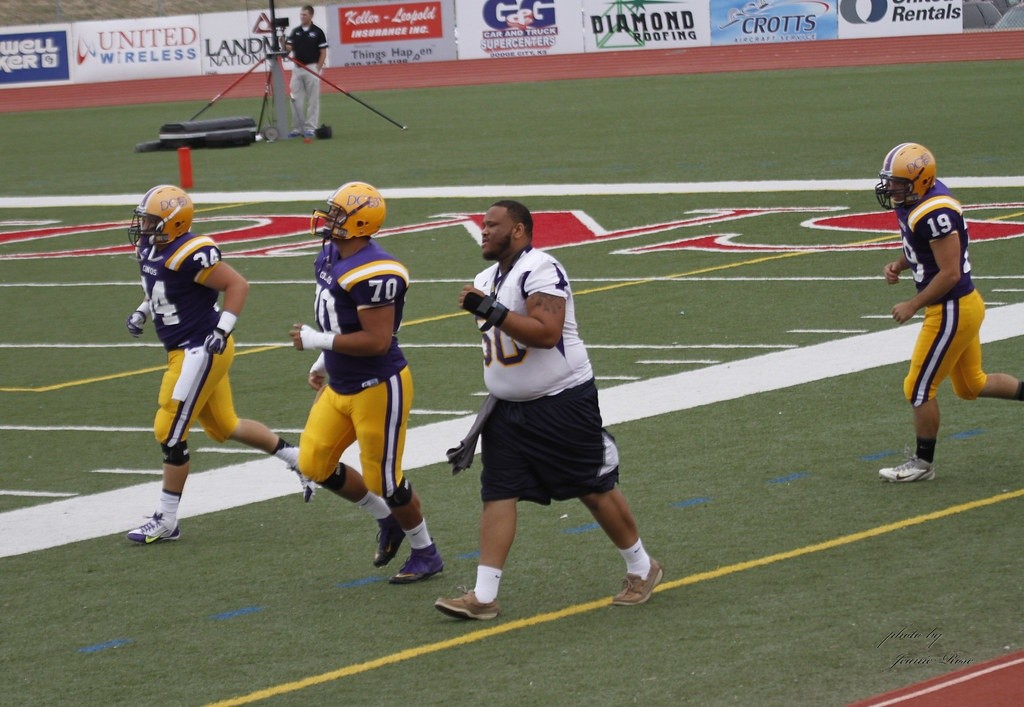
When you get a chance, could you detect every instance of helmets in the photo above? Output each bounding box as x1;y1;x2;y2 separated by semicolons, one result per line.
875;142;937;210
310;182;387;241
129;184;193;248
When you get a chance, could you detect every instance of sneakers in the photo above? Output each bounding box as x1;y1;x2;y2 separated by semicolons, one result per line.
612;559;662;605
388;550;443;584
287;460;316;503
288;132;314;137
434;585;499;620
878;454;936;482
125;512;181;544
372;520;406;568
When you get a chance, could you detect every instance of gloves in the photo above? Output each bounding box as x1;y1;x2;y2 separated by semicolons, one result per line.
204;327;227;355
126;311;146;338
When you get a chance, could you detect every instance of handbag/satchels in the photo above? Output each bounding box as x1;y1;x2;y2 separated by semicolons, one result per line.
314;124;332;139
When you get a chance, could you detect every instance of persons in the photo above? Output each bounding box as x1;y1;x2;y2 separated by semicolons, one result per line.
284;5;331;139
127;185;317;543
876;142;1024;484
288;180;445;585
433;199;663;621
